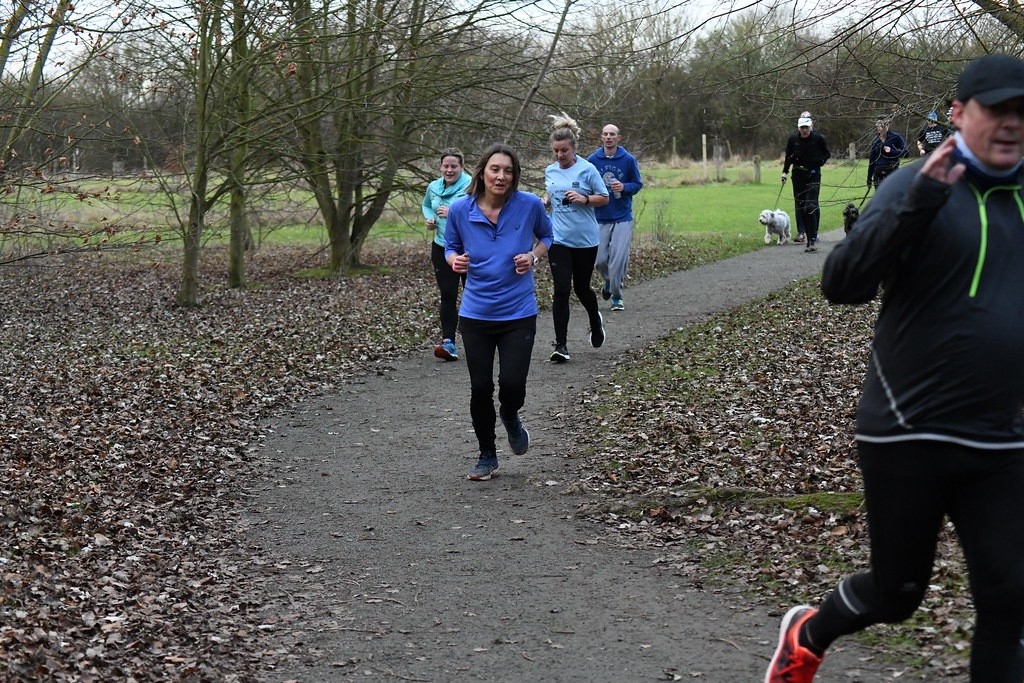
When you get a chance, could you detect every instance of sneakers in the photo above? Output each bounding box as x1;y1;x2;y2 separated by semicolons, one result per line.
466;450;500;480
550;342;570;361
601;279;612;300
434;338;459;361
764;603;826;683
585;311;606;348
499;404;530;455
610;296;625;311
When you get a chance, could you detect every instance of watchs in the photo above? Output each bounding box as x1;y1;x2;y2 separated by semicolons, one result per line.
527;252;539;266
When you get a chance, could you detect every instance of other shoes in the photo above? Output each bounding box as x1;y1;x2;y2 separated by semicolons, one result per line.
795;232;805;243
804;238;817;252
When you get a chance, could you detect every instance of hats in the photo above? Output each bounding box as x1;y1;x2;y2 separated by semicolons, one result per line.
925;112;938;121
797;117;812;128
946;107;954;115
957;54;1024;107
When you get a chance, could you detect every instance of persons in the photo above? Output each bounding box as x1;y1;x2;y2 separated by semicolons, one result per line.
917;112;950;158
781;118;831;251
765;54;1024;683
444;144;555;478
540;120;610;362
866;115;910;191
422;148;474;361
587;124;643;311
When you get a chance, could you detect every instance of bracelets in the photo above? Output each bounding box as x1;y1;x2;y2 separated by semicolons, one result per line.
584;195;589;204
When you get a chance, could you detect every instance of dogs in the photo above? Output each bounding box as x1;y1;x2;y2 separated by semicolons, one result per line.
758;208;791;245
843;204;859;235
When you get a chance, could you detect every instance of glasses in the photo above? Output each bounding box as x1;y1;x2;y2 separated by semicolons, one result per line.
946;114;951;117
